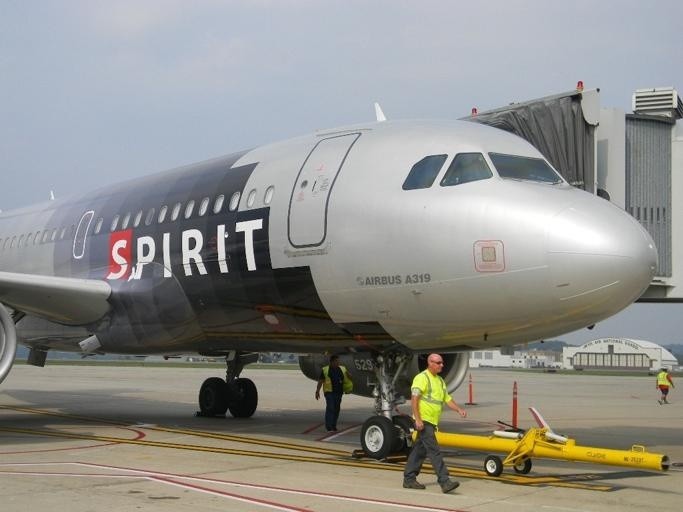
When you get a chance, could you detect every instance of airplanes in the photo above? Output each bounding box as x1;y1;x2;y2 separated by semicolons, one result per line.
0;89;664;460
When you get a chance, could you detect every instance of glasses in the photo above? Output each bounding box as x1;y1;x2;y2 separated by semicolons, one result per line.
433;361;445;366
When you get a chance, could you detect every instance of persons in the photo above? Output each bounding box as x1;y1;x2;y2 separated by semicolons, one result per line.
403;354;467;493
656;367;675;404
315;355;352;433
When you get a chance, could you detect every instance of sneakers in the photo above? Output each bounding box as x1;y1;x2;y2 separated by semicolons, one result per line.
441;479;460;493
325;424;338;433
403;481;425;489
